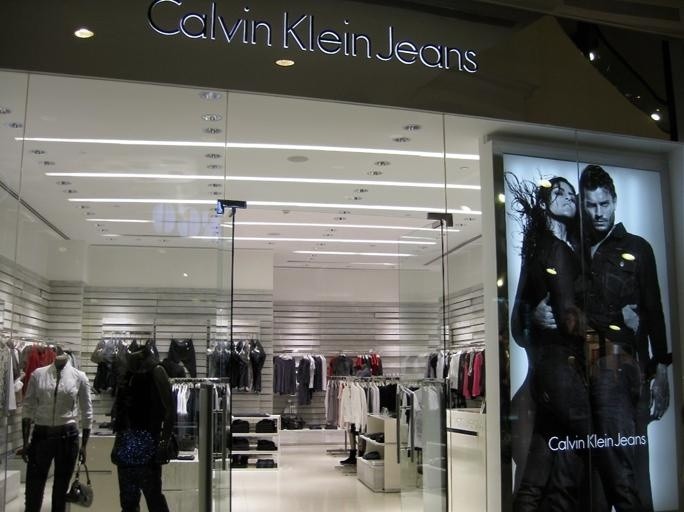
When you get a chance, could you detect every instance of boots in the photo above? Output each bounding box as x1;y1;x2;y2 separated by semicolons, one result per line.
340;449;357;464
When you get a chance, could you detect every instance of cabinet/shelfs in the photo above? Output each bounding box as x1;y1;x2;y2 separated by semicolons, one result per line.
355;412;408;494
225;412;282;470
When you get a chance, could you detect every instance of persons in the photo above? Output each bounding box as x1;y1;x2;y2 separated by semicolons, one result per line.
533;163;673;512
12;354;94;512
336;360;371;465
108;346;176;511
504;171;640;511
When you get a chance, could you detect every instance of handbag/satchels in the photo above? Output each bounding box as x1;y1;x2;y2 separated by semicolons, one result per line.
65;461;95;507
